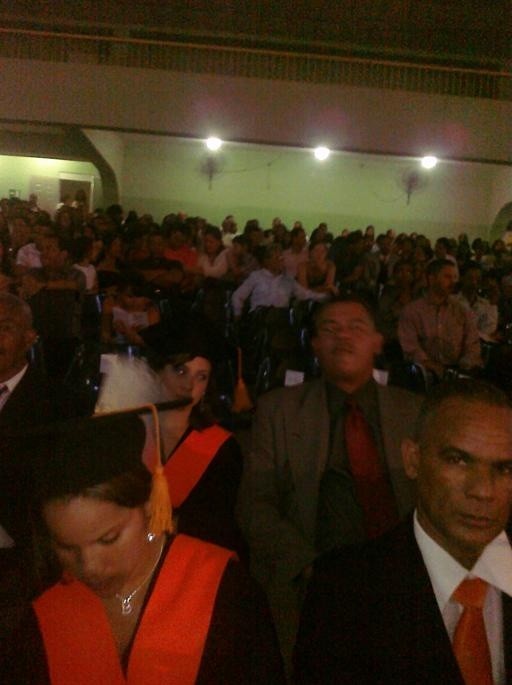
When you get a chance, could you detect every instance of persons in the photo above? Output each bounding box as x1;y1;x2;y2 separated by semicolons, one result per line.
294;375;511;685
0;396;285;685
0;295;94;598
130;311;249;567
234;295;424;671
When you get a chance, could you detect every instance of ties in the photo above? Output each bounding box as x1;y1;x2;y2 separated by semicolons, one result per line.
0;383;10;406
453;579;493;684
343;397;399;538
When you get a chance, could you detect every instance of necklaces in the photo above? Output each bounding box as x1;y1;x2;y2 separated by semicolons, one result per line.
115;543;163;615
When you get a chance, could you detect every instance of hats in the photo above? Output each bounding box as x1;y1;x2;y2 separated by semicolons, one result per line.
137;314;252;415
2;398;194;541
